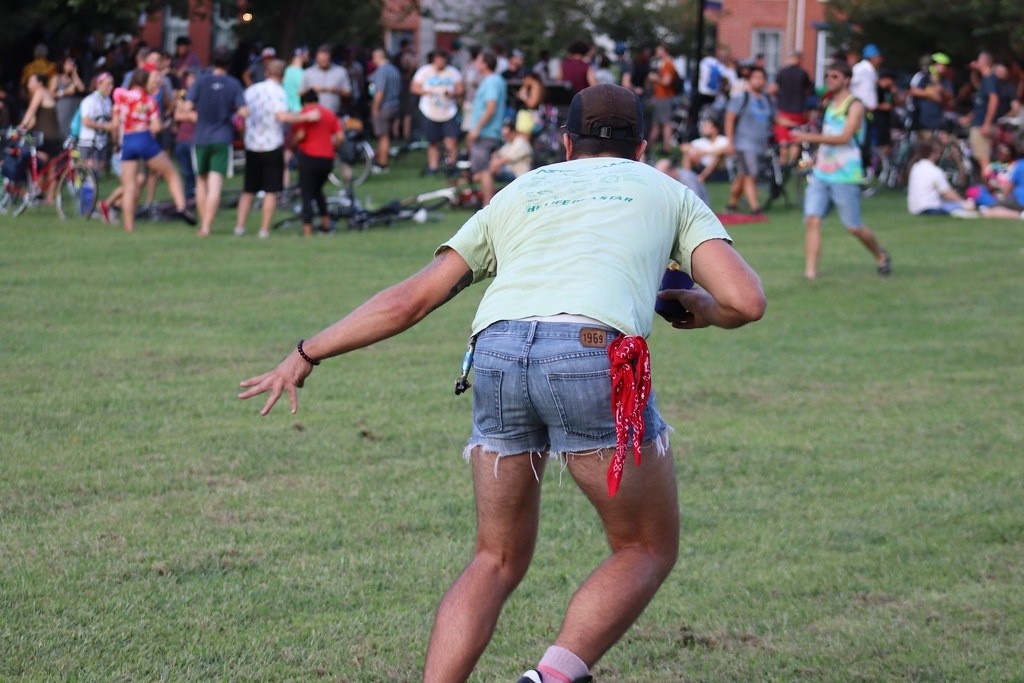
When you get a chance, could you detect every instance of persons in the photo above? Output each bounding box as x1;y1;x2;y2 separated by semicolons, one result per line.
0;37;1024;280
237;84;767;683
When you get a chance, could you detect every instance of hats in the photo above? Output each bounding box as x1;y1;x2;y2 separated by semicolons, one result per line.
863;45;878;60
561;83;644;139
931;53;951;68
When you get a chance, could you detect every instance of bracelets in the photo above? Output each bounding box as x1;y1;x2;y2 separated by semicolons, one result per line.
297;340;320;365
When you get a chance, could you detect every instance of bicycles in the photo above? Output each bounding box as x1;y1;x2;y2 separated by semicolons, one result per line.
0;128;99;222
137;100;484;232
724;106;920;212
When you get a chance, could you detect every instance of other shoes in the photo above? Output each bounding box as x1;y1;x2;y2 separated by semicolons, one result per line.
99;201;110;222
174;209;196;225
878;250;890;275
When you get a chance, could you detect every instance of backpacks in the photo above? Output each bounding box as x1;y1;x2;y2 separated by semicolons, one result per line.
845;99;877;179
